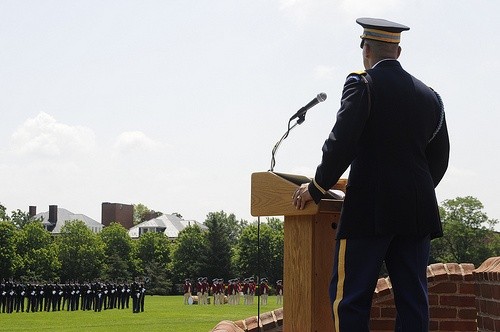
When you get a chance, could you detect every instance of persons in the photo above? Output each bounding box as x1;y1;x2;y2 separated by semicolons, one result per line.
242;277;257;304
117;280;125;310
292;17;450;332
0;277;117;314
259;278;270;305
196;277;210;305
212;278;226;305
227;277;242;305
275;280;283;305
184;278;192;305
131;277;141;313
138;278;146;313
124;280;131;308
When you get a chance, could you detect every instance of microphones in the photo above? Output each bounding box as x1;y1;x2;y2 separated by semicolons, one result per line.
291;92;328;119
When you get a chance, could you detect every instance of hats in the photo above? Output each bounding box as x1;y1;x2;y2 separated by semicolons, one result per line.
230;277;240;283
185;277;190;282
276;280;282;283
212;277;224;282
260;278;268;283
198;276;208;282
244;276;255;283
355;17;411;49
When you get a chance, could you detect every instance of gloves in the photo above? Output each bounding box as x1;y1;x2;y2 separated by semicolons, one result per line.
2;289;130;298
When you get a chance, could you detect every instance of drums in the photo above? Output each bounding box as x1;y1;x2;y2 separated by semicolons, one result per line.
203;297;210;304
224;295;229;304
187;296;199;305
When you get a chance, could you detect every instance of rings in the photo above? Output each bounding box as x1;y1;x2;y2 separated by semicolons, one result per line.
297;196;300;200
